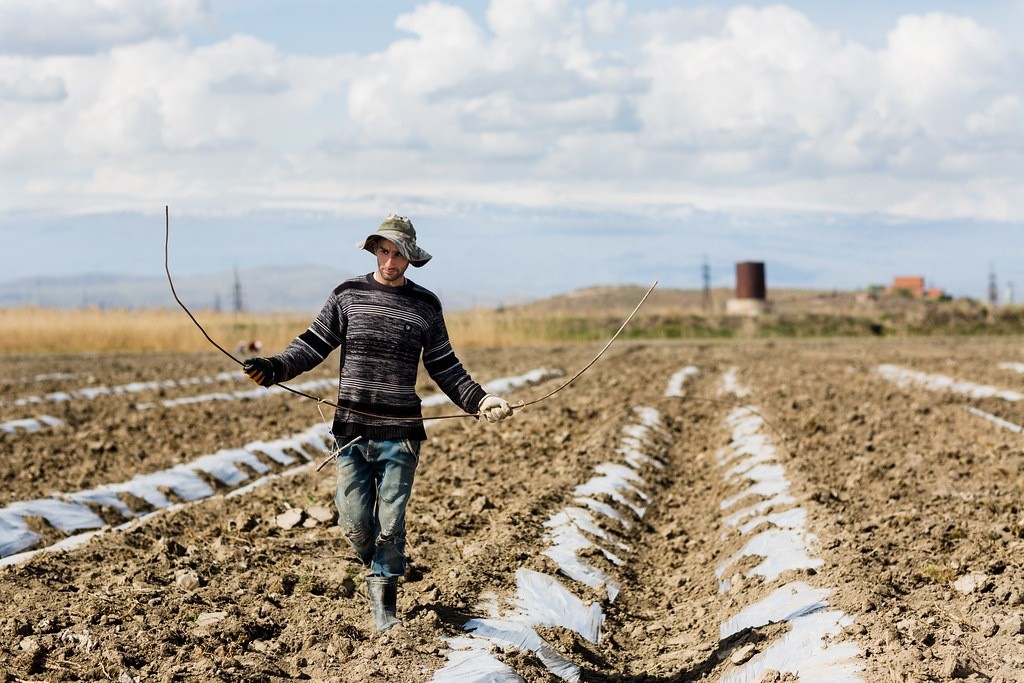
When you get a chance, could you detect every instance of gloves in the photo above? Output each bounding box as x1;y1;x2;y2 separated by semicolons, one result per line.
477;394;513;423
242;358;276;387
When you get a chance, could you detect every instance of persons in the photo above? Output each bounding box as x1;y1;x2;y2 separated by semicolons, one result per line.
244;214;511;635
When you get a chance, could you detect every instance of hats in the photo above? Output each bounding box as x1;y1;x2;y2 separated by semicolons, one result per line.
356;213;432;268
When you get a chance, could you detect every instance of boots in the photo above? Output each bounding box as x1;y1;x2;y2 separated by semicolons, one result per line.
353;527;375;569
365;574;403;636
